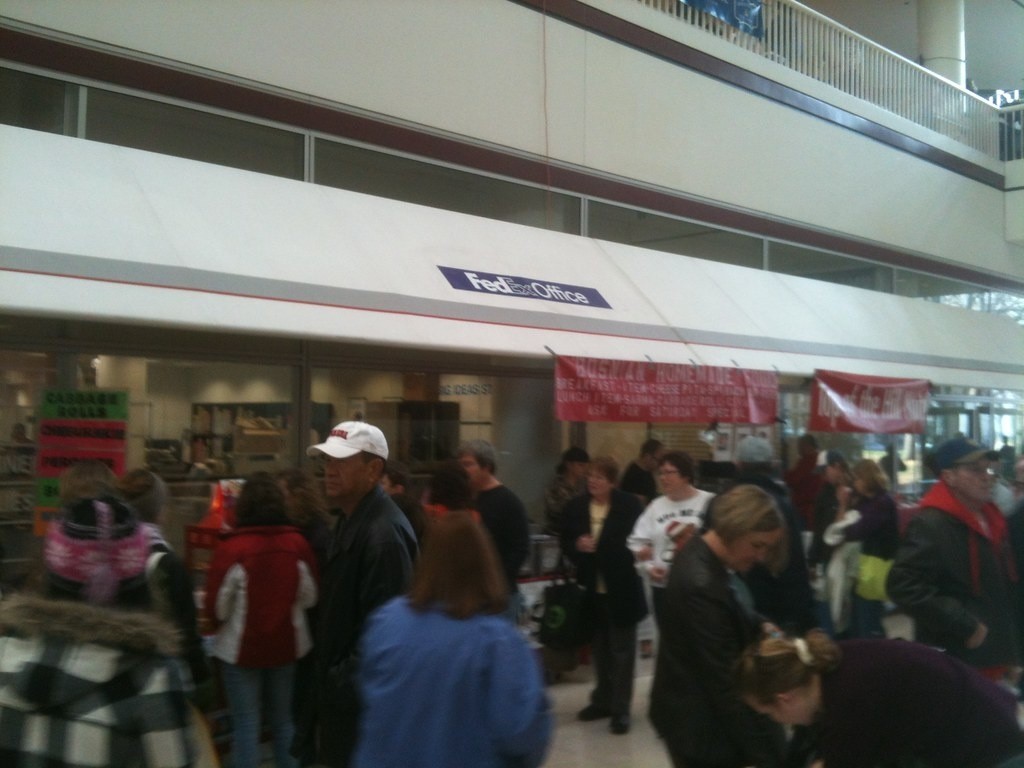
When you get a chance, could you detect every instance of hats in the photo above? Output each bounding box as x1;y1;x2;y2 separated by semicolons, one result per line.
561;446;593;464
925;434;999;475
120;469;171;514
811;448;845;472
41;498;147;587
736;435;772;463
306;421;388;459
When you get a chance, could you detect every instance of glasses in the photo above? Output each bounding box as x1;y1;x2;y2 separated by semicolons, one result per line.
657;468;679;477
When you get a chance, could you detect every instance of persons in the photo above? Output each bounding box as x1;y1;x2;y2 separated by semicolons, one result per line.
0;495;218;768
117;469;218;737
349;511;553;768
60;458;118;501
203;417;529;768
737;629;1024;768
548;436;1024;768
10;423;36;530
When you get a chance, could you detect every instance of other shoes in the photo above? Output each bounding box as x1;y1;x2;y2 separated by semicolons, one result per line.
610;709;630;735
577;703;610;721
